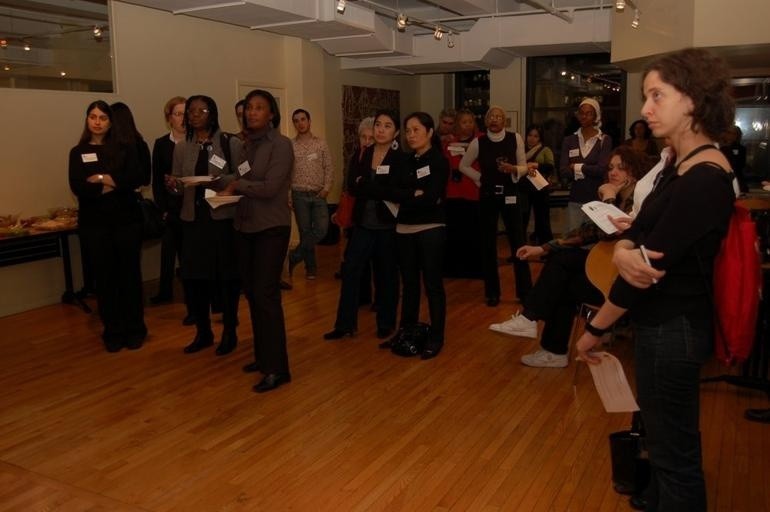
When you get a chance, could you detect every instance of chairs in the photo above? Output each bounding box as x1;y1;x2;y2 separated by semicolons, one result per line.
565;241;620;364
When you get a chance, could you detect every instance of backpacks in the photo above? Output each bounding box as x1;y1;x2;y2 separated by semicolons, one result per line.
679;161;761;368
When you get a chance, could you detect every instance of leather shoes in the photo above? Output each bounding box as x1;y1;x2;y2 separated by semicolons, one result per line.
99;321;123;353
249;369;292;393
124;319;149;350
377;325;405;352
242;359;265;373
612;485;635;496
145;288;172;310
180;310;196;328
744;405;769;424
628;493;657;511
418;334;445;360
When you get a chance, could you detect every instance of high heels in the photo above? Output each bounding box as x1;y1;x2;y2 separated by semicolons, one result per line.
485;289;501;308
321;324;359;341
375;324;391;341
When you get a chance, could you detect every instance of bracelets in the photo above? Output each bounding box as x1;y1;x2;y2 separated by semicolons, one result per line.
585;322;604;337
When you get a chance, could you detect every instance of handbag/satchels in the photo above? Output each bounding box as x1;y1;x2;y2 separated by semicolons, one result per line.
608;398;654;488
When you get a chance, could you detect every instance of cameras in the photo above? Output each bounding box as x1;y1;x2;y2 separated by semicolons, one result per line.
452;170;463;181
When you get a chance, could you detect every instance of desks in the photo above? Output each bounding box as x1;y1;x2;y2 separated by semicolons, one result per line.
0;215;94;315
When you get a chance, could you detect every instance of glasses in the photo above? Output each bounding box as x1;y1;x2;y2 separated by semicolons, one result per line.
169;110;185;116
359;132;375;141
485;115;504;122
187;107;209;113
579;110;593;116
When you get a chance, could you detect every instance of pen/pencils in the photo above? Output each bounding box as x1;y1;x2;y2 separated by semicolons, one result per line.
639;244;660;289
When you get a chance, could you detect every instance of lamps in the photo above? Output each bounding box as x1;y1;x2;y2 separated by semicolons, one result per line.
337;0;345;11
434;27;443;39
397;12;406;31
616;0;627;12
632;10;640;29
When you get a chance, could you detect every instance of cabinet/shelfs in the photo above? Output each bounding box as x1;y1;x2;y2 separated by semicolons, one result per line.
455;70;491;132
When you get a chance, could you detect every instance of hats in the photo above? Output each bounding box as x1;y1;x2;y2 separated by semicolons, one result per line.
579;97;601;123
481;103;509;123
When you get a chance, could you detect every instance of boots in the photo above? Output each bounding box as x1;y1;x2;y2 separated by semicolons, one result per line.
183;314;216;355
215;318;238;356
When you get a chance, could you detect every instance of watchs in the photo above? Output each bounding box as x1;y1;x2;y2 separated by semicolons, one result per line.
99;173;103;184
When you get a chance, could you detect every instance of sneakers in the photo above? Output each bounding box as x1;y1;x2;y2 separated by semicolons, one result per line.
520;348;569;368
488;309;538;339
285;246;301;281
304;265;318;281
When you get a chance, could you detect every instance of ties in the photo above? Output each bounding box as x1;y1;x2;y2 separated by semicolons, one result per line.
193;139;210;222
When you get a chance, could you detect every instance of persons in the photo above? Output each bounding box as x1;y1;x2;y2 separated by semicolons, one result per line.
215;89;294;393
332;117;377;282
324;111;404;339
503;124;553;264
69;101;113;298
164;95;245;356
560;96;614;233
574;50;740;512
97;102;152;352
442;110;484;280
435;107;457;148
377;112;448;359
489;146;653;368
152;96;200;304
288;109;335;279
618;120;661;168
458;105;533;308
